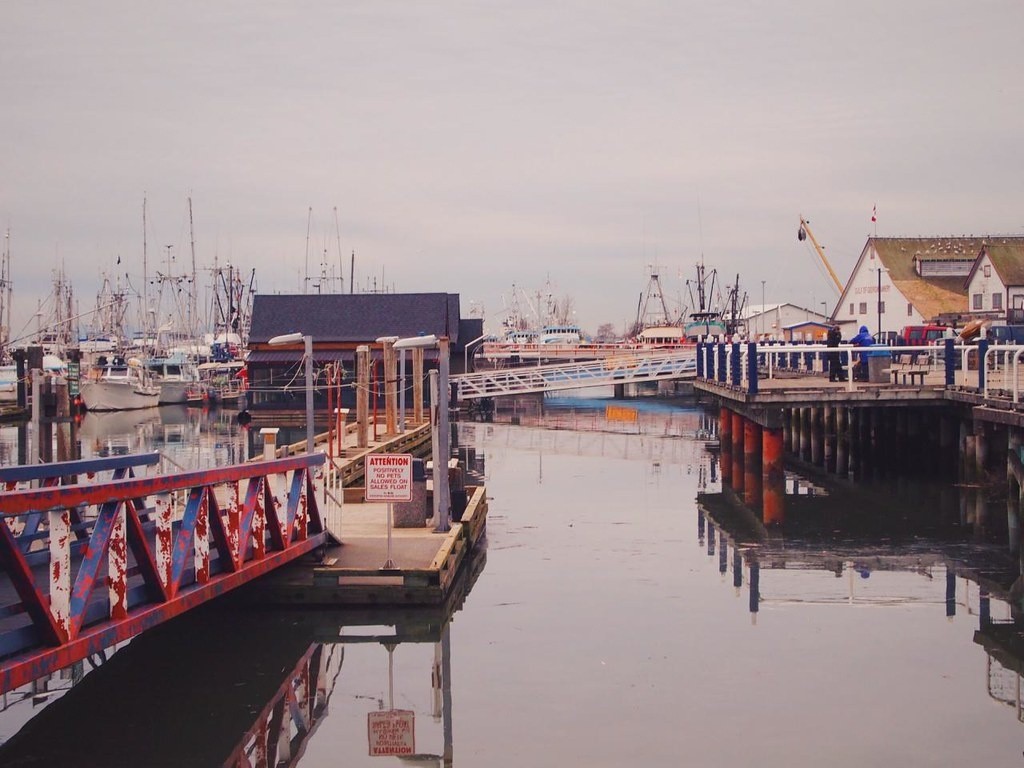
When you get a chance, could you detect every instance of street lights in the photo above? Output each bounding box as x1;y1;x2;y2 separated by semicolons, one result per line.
821;301;828;322
267;331;316;485
391;333;453;533
375;335;406;434
761;280;766;333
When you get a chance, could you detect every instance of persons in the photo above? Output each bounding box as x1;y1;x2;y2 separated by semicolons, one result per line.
849;325;875;381
827;326;848;382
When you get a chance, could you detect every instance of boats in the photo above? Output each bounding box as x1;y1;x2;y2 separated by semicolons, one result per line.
633;250;729;345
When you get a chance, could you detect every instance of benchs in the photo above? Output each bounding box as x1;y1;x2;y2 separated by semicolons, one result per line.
882;354;932;384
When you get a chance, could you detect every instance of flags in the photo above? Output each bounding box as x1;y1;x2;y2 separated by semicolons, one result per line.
871;206;878;222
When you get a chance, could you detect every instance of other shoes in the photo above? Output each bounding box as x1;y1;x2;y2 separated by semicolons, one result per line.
839;378;849;381
830;379;839;382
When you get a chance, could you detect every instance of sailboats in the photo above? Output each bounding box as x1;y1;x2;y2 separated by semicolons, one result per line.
499;272;580;346
0;188;252;430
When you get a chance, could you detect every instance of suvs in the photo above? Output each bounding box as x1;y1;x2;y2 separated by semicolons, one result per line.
869;320;1024;365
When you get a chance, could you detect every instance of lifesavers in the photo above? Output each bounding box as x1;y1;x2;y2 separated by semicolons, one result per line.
230;346;237;355
238;412;250;424
